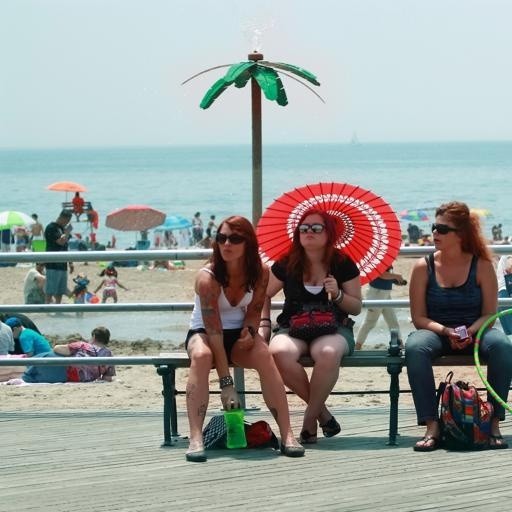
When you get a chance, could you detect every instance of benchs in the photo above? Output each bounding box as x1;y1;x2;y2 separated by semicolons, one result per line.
152;352;487;450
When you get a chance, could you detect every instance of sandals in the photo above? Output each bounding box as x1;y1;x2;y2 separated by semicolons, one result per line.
185;446;206;462
280;439;305;458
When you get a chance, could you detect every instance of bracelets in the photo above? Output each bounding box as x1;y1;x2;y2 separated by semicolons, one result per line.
259;318;271;323
259;325;272;328
332;289;344;305
442;326;447;335
219;376;233;388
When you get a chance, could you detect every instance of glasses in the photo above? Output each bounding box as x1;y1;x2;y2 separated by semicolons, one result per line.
215;232;247;245
298;223;328;234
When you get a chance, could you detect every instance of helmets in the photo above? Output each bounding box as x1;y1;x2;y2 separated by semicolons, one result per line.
432;222;461;235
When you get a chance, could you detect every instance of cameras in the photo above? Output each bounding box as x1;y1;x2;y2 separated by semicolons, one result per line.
394;279;407;285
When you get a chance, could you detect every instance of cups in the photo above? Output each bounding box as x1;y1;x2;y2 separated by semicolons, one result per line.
390;328;398;347
453;325;467;342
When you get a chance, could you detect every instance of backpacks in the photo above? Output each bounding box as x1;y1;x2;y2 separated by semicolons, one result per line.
439;377;494;450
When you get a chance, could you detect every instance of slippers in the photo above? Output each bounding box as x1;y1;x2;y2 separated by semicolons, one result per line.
412;433;439;452
319;416;341;438
490;430;508;450
300;429;318;444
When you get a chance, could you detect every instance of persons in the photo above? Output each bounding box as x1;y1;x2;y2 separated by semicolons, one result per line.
141;212;219;249
355;265;407;349
403;222;435;247
182;216;305;461
492;224;512;244
0;192;126;385
404;202;512;451
495;255;511;334
256;210;361;445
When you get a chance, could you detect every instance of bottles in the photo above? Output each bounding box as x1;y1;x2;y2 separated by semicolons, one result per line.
224;402;248;449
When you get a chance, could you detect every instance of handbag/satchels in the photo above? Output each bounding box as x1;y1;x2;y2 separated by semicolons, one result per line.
289;302;339;336
205;414;280;451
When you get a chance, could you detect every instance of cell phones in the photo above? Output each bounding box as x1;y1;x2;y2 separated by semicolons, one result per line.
455;325;469;342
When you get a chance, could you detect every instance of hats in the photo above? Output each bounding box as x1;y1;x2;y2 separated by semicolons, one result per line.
4;317;22;328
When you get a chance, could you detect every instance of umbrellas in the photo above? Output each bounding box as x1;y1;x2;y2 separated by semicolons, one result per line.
106;207;166;233
48;182;87;202
252;182;402;285
400;208;430;221
